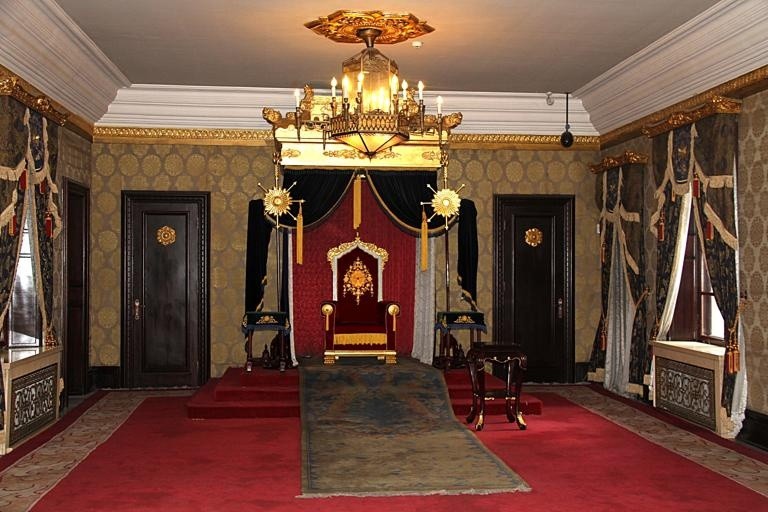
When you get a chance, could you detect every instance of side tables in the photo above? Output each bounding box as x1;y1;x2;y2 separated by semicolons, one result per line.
437;311;485;371
242;310;288;373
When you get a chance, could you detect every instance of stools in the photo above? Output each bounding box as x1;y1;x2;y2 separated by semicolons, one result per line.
463;344;529;432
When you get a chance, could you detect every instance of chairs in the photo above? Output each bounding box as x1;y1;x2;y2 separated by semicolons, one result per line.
319;232;400;365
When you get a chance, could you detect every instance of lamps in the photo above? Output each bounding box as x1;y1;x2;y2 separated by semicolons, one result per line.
294;8;446;164
